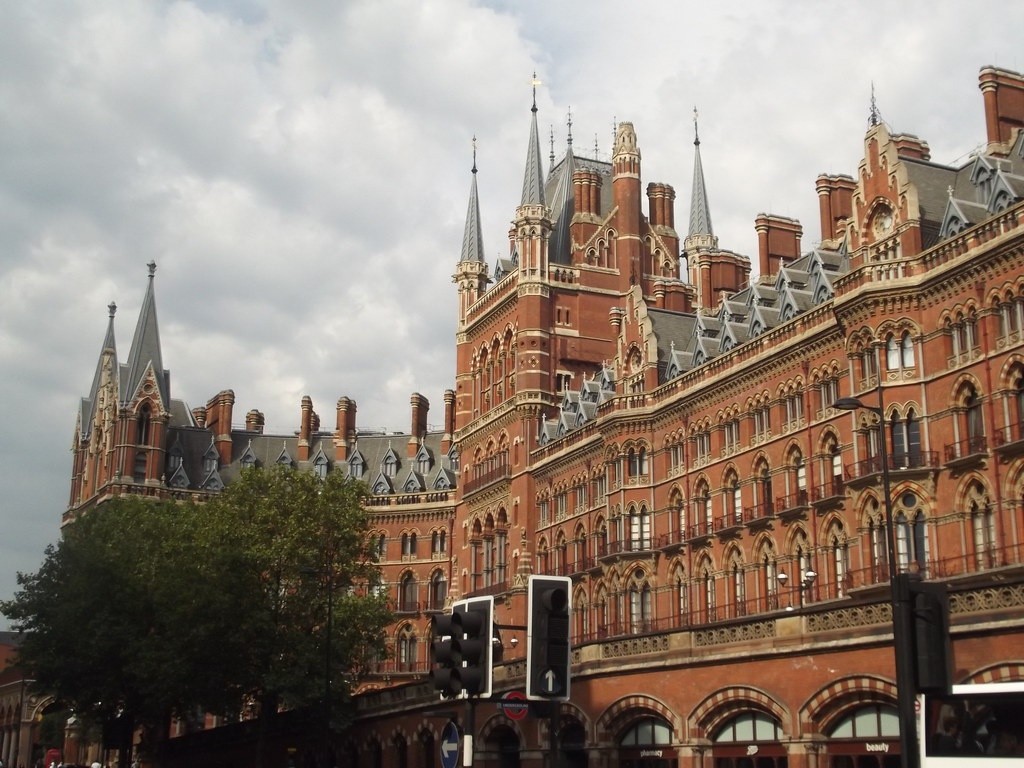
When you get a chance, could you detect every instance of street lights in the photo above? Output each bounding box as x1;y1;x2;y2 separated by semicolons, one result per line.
832;394;931;768
300;569;339;712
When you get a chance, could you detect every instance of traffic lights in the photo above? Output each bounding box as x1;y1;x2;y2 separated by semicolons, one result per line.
423;597;493;702
529;574;569;702
